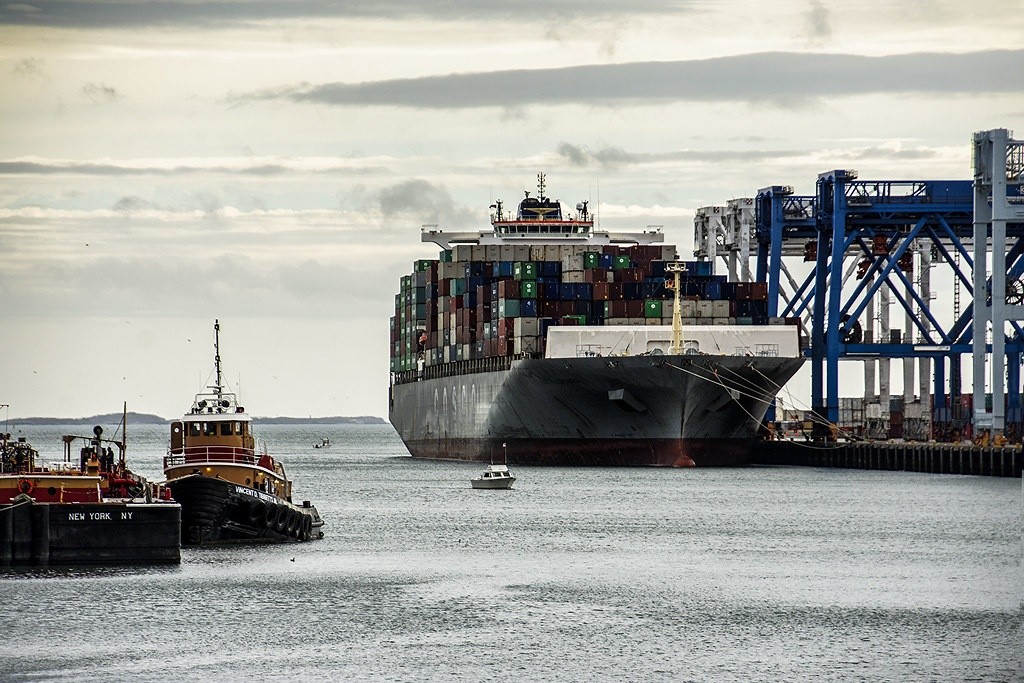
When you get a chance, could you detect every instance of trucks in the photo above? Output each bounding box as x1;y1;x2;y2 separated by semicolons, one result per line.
47;461;72;471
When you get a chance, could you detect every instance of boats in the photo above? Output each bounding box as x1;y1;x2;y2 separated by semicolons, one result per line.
312;437;331;449
469;464;515;489
163;318;325;544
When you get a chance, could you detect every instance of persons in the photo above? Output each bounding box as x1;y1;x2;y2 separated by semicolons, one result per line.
99;448;107;472
106;448;114;472
16;450;25;475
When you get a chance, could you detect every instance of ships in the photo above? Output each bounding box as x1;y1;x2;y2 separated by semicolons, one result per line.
387;172;802;468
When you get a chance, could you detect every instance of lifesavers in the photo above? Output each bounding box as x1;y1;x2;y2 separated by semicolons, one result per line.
265;501;312;543
246;499;265;521
17;479;34;493
